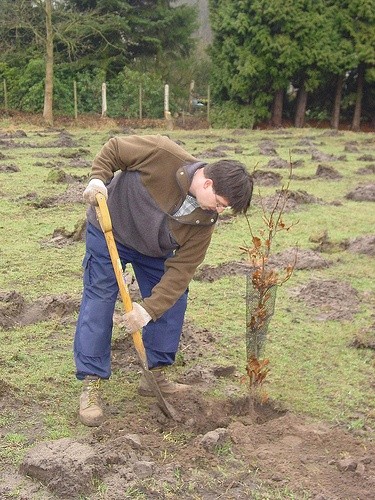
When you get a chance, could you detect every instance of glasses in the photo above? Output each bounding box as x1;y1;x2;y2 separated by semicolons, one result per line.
209;178;227;208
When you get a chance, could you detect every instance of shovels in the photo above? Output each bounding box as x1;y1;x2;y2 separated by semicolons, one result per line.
93;193;176;422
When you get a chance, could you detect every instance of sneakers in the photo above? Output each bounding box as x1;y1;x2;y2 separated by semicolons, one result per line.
79;375;103;427
138;367;192;397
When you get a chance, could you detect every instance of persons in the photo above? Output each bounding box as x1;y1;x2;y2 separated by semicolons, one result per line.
74;135;254;428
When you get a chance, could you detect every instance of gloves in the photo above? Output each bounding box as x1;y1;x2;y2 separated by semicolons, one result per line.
118;302;152;334
83;179;108;206
